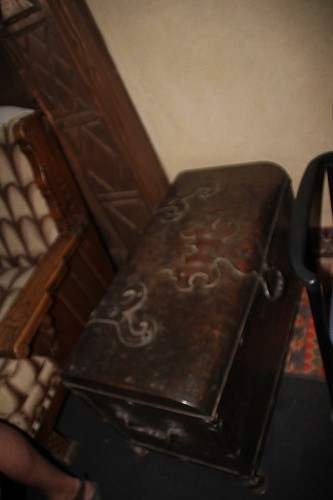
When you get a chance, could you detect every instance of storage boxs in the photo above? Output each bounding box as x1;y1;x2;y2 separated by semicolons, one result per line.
60;161;306;480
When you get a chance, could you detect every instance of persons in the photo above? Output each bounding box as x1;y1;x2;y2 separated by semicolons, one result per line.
0;420;103;500
